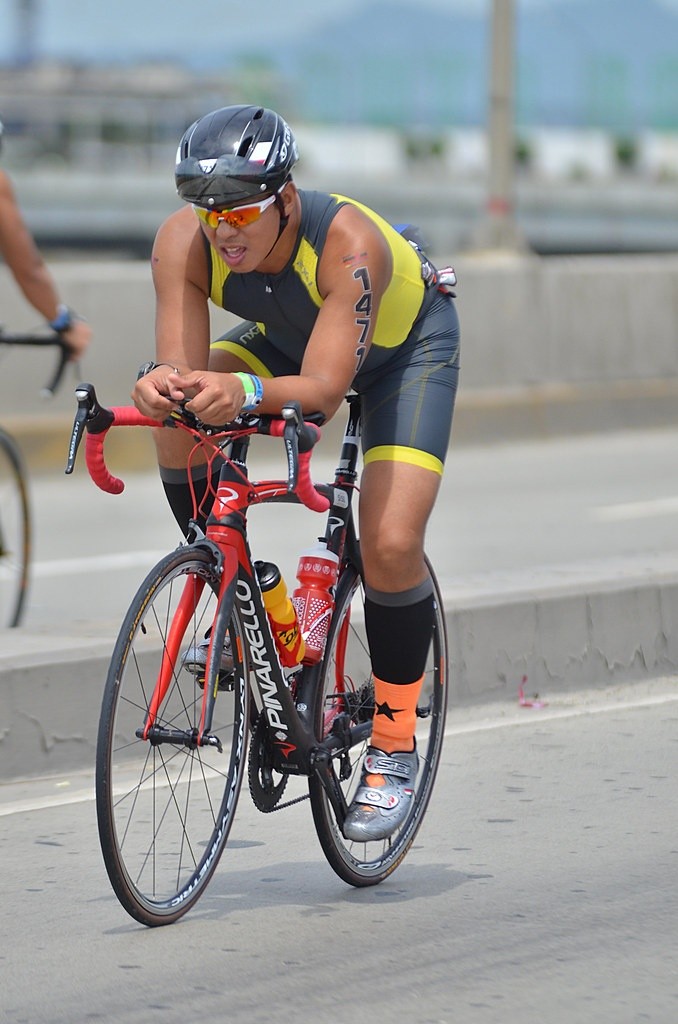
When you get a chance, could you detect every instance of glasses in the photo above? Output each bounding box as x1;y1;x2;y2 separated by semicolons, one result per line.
191;180;289;228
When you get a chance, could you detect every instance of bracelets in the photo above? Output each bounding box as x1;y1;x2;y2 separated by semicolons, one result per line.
231;372;263;412
50;305;72;331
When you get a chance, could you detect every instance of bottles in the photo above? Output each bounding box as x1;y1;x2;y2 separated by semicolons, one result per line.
254;561;305;666
293;537;340;665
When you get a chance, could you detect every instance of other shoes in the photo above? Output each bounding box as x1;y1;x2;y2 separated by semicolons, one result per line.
343;735;420;841
181;631;234;673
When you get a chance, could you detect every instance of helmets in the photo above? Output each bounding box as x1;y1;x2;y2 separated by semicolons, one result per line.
174;104;295;211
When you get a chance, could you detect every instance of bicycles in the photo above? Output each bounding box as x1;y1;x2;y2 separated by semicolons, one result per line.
65;380;451;929
1;329;76;634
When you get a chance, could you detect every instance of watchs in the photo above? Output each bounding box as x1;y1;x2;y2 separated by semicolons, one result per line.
137;361;180;381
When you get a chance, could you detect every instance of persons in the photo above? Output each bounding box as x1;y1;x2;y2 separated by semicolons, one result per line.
131;105;460;842
0;121;91;361
